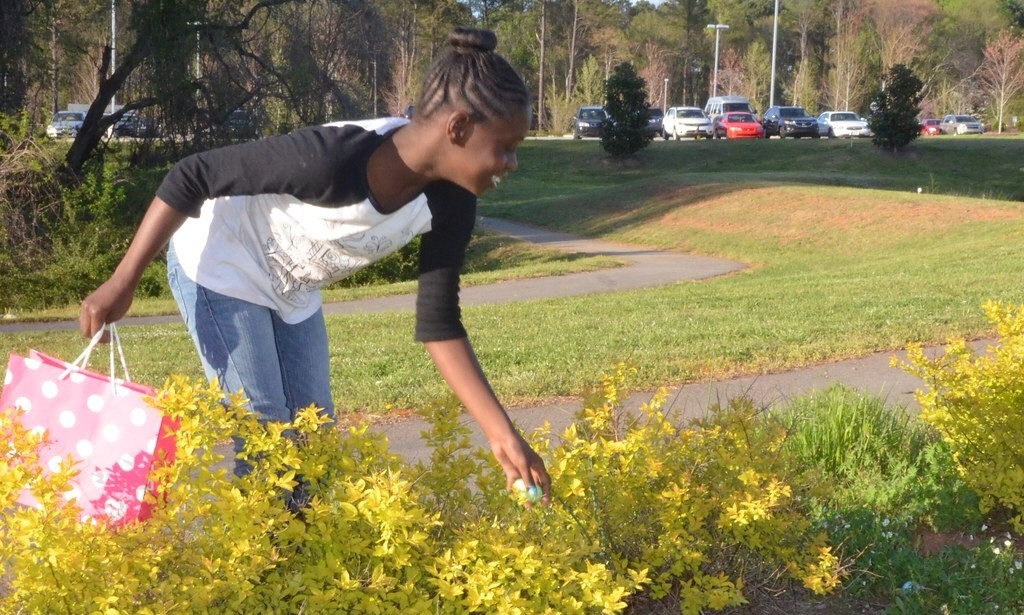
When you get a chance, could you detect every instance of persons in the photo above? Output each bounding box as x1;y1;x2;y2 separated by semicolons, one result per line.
77;27;553;535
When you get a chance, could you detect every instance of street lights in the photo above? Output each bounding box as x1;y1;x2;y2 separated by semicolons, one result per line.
187;20;208;109
707;23;730;97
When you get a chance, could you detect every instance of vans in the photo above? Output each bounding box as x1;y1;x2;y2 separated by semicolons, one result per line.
703;94;757;121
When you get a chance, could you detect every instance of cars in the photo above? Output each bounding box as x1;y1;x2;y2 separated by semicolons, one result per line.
637;106;666;139
660;106;714;142
111;105;263;139
573;104;616;140
919;119;944;135
939;114;985;135
713;111;764;139
45;110;87;139
816;111;871;138
760;105;819;139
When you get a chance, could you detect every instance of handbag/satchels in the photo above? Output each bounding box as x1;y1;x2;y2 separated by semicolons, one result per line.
0;322;181;534
